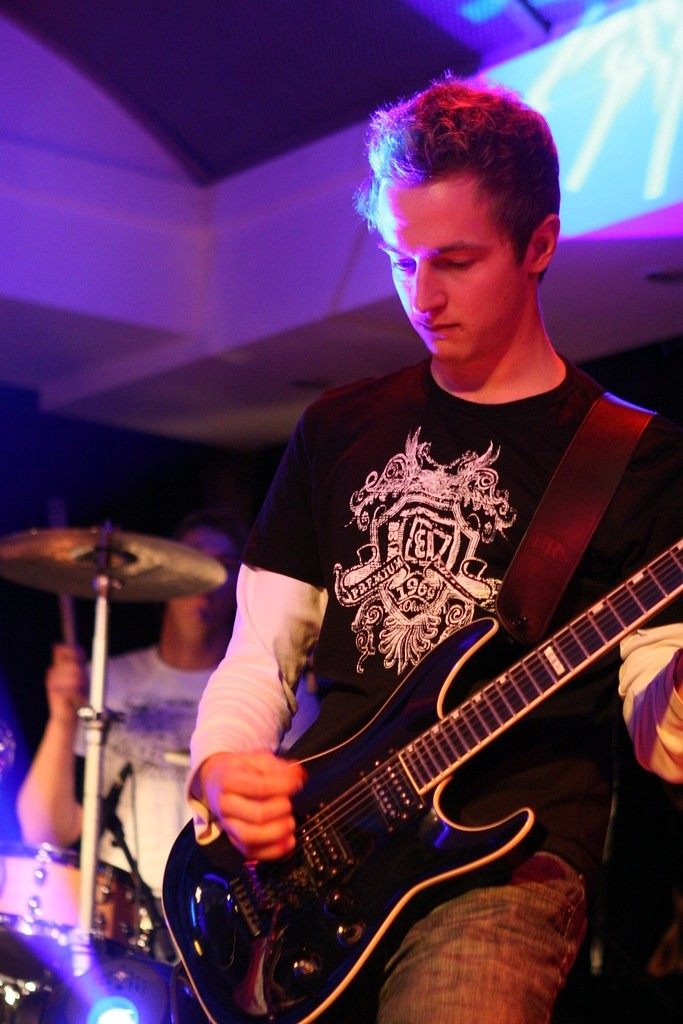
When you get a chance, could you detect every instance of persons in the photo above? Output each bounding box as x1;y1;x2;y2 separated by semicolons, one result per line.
183;70;683;1024
17;503;326;901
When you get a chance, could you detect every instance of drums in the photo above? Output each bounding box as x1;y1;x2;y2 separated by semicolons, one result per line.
0;837;155;995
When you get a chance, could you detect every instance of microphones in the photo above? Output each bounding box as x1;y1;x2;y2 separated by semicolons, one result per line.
97;767;130;837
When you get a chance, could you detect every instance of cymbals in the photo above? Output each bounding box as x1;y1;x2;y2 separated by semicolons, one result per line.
0;516;228;603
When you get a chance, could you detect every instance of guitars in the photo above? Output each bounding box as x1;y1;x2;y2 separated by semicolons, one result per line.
158;534;683;1024
